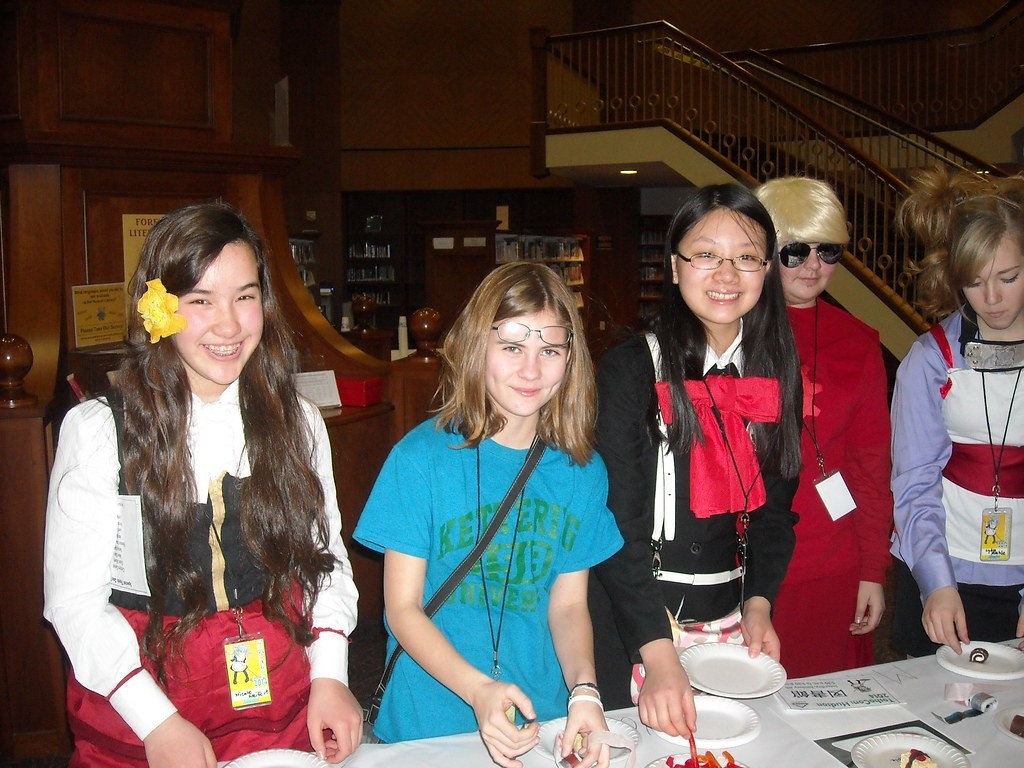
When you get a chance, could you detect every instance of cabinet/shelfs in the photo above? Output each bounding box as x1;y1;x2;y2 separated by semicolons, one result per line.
273;220;594;361
638;231;670;329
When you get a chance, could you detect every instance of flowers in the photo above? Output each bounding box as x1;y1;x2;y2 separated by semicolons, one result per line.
136;278;187;344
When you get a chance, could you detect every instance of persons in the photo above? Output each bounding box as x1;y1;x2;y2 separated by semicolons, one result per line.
750;176;913;680
351;262;626;768
40;200;365;768
585;181;804;741
889;163;1024;660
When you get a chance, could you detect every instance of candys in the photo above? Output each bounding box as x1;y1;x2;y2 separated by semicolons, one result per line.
665;732;745;768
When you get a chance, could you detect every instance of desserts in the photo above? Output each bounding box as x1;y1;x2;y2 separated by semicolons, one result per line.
900;748;938;768
970;647;989;663
1010;715;1024;737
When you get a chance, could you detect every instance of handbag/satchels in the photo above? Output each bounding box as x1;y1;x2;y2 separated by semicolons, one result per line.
346;623;389;722
630;603;748;707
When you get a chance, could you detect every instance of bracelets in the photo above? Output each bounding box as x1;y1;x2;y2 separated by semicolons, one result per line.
568;683;604;715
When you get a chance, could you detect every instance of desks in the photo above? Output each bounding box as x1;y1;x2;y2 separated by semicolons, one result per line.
210;636;1024;768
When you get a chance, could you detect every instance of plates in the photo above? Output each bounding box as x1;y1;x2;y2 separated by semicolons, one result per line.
644;753;749;768
936;640;1024;680
994;705;1024;744
850;733;973;768
532;716;642;763
651;694;761;749
221;748;329;768
680;642;787;698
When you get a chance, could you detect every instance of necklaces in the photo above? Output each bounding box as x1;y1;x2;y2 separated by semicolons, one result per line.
472;432;542;681
693;353;784;571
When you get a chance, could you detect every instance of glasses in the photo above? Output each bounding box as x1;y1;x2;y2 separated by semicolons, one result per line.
491;322;574;346
777;242;843;268
675;252;770;272
